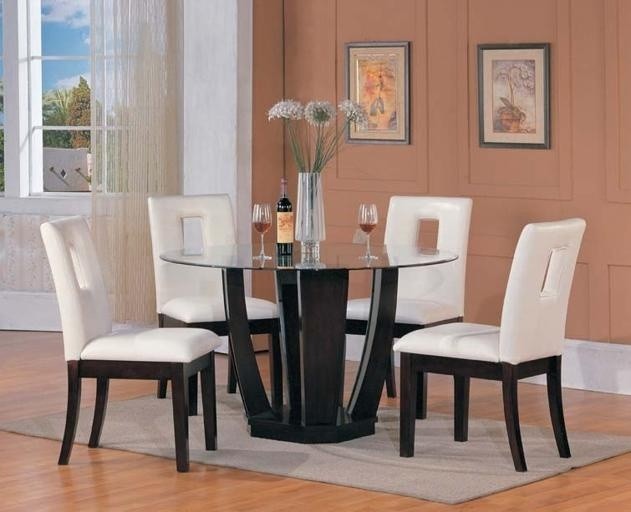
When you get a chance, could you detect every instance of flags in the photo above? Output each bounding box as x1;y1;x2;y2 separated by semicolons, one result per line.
295;173;326;269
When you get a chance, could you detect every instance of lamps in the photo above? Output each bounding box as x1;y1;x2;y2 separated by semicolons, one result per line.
147;194;282;417
39;216;223;473
392;217;586;471
345;196;472;419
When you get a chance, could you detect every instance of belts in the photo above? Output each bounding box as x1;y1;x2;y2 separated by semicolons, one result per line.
253;203;273;261
358;204;378;260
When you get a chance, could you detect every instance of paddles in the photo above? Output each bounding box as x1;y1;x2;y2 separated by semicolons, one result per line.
0;384;631;504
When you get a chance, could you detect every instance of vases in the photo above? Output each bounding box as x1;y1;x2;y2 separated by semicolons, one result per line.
267;97;360;232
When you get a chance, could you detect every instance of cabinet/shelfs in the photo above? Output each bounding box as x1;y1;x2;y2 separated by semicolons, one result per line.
277;178;295;258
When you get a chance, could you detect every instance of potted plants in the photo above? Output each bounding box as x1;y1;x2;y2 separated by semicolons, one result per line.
344;41;410;144
476;43;550;150
